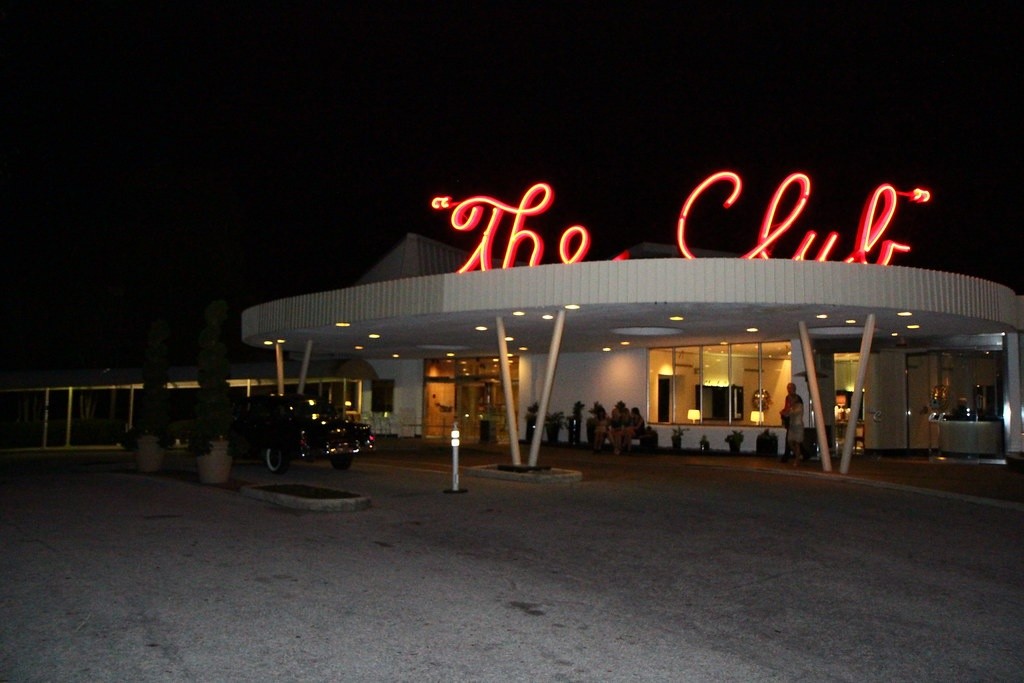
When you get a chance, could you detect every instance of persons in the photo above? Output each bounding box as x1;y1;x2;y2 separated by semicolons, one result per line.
593;408;644;455
957;397;972;420
785;383;805;467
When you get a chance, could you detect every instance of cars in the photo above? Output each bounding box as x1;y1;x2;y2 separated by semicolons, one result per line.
185;395;375;473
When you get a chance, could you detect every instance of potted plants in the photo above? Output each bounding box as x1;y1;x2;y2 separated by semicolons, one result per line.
699;435;709;450
567;401;585;445
670;426;690;449
524;402;539;441
756;430;778;457
119;317;176;473
725;430;745;453
190;299;250;485
545;411;568;441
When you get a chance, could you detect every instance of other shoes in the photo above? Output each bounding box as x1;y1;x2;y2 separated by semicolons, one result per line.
794;463;801;467
614;450;620;456
592;449;601;454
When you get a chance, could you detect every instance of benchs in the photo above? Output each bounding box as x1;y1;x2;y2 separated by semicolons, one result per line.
939;421;1002;454
635;432;658;450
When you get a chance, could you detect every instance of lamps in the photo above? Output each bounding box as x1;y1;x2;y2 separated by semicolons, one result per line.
658;350;674;376
750;410;765;426
687;409;701;423
